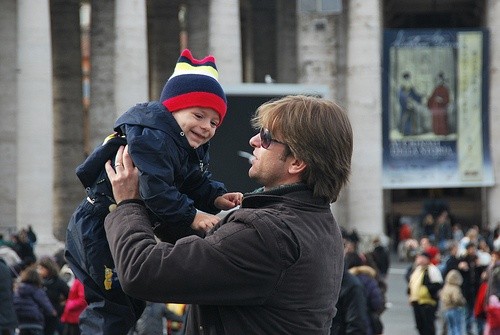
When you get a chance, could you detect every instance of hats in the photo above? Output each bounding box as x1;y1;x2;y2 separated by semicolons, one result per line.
159;49;227;128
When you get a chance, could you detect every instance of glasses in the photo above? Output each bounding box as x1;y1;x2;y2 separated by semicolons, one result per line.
259;127;291;149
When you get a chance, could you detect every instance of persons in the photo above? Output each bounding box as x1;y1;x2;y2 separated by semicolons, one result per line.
106;94;354;335
337;209;500;334
64;49;244;335
0;227;188;335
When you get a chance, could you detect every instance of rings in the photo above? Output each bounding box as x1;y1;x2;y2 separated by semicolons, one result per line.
115;163;122;167
